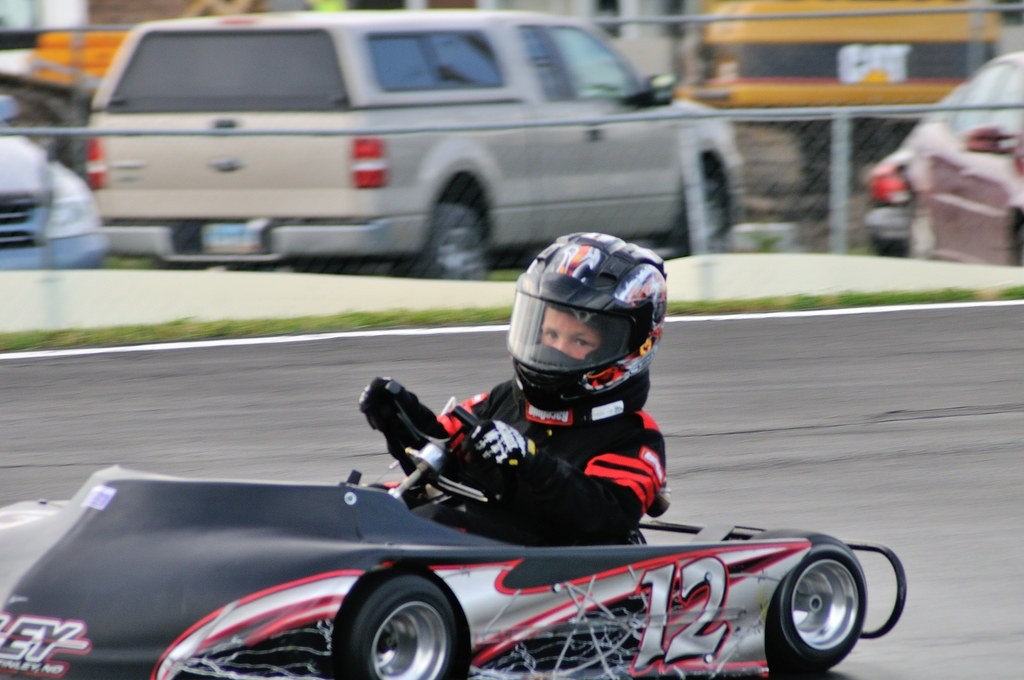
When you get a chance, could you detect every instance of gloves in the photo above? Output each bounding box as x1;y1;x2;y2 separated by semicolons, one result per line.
469;418;558;485
359;377;436;457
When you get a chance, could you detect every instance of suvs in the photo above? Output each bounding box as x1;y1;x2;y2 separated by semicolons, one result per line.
82;8;743;280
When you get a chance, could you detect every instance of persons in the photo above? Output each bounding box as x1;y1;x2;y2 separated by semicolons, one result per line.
361;231;667;544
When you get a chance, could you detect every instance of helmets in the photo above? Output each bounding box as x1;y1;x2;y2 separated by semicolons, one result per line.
505;232;666;425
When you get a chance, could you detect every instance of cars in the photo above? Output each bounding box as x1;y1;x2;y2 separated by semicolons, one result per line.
864;51;1024;267
1;93;106;273
